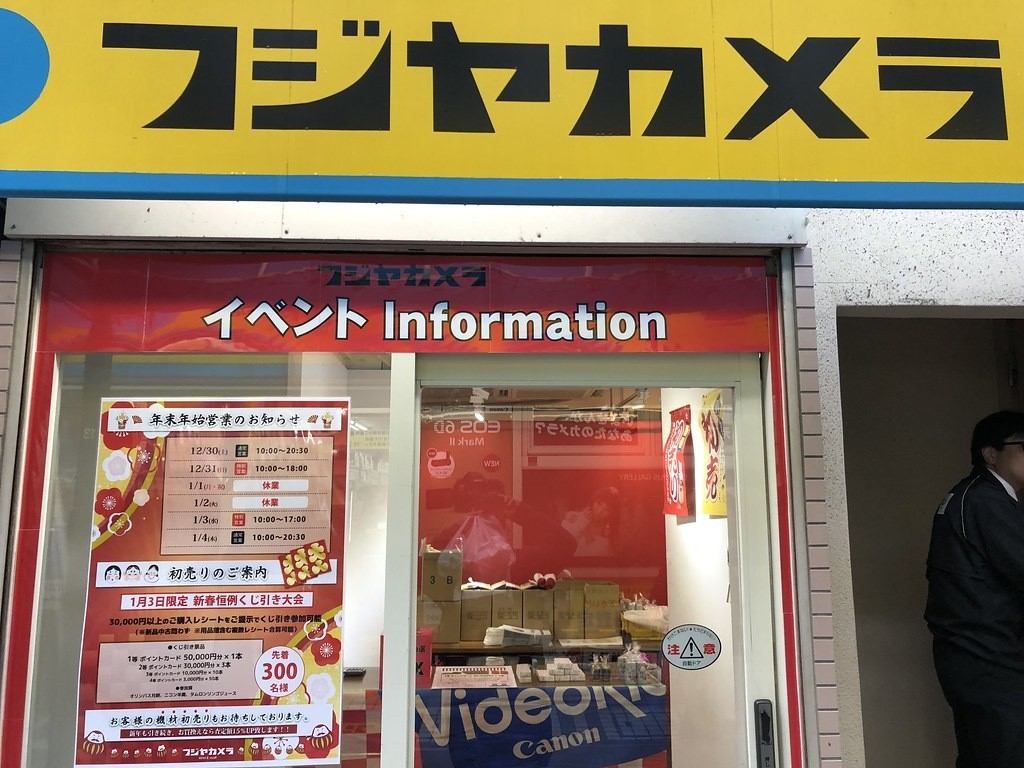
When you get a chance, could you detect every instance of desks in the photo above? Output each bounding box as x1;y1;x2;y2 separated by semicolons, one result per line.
414;637;667;768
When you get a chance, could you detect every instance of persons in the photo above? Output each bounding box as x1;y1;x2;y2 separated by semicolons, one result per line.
438;471;628;596
923;410;1024;768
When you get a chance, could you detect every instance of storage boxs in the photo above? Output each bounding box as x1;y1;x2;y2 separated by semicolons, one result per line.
417;537;620;645
